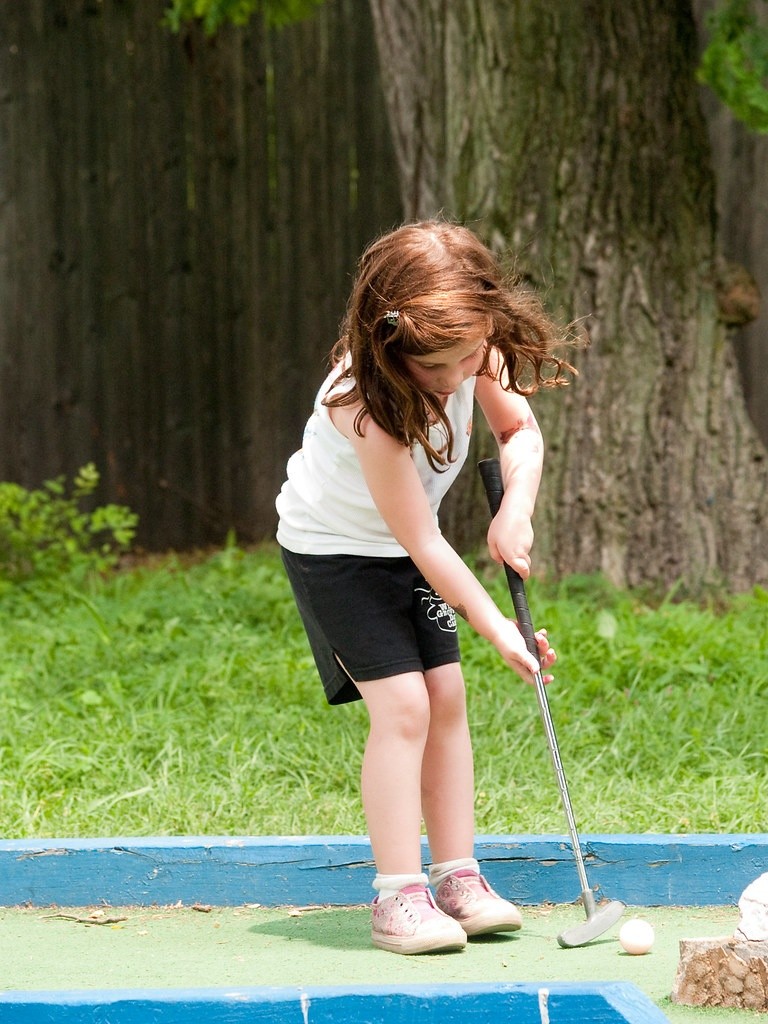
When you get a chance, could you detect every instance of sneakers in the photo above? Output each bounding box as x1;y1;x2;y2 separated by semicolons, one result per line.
370;868;523;956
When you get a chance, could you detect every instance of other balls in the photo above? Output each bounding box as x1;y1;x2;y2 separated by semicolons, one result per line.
617;916;656;955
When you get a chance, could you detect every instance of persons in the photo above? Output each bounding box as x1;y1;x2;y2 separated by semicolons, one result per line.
277;221;589;953
